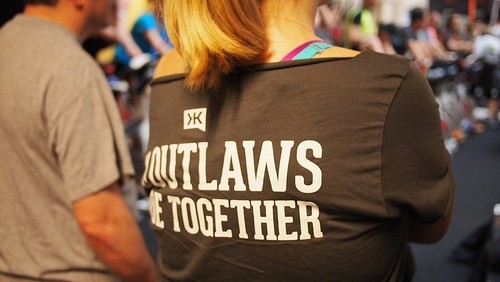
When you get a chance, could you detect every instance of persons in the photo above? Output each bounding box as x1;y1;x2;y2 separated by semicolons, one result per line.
86;1;500;156
141;0;456;282
0;0;162;282
